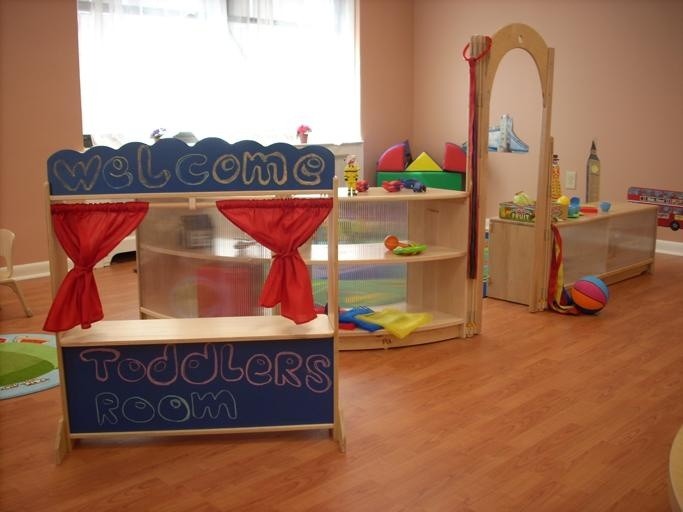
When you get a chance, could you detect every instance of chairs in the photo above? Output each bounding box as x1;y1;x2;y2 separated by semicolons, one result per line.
0;228;35;317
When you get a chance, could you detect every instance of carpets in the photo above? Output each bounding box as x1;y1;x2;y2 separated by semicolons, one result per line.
0;334;60;401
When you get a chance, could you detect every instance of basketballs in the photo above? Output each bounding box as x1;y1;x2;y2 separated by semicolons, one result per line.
571;275;609;314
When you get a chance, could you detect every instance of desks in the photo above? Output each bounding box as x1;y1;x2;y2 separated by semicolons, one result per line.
488;200;658;310
666;425;683;512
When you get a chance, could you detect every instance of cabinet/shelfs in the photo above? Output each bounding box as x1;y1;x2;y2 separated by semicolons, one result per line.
136;184;472;351
41;174;346;466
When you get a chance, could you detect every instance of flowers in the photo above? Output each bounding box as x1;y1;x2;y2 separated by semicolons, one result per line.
150;128;166;138
296;124;312;135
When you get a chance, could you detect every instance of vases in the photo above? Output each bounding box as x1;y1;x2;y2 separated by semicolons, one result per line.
299;134;308;144
